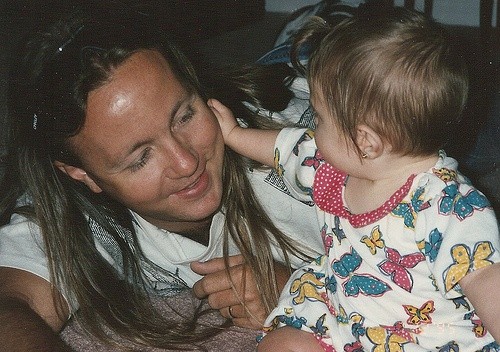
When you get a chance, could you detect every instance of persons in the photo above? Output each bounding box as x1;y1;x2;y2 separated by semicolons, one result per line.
205;7;500;352
0;5;356;352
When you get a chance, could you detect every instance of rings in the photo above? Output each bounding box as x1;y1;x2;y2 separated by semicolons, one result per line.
228;306;237;321
